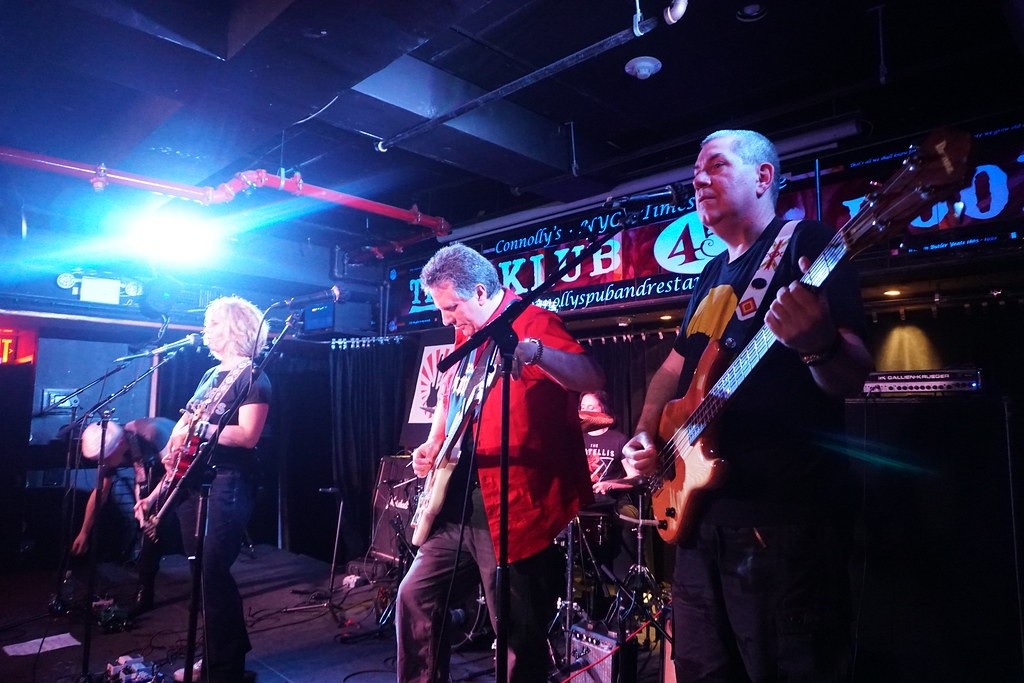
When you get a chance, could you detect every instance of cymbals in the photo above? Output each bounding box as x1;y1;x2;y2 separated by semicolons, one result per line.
577;410;615;434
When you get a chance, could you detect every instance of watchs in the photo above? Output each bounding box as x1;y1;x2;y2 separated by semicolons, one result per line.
799;327;842;366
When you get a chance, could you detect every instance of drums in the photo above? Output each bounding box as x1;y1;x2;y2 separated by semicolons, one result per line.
553;511;609;545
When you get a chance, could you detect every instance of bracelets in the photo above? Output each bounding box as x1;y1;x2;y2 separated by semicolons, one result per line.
524;337;543;366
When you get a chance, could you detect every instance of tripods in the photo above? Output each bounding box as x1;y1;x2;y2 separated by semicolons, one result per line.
0;358;138;633
599;490;666;627
280;487;349;628
547;520;598;641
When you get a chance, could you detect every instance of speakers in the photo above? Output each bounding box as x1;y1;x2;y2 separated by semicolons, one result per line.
839;395;1024;683
569;625;638;683
370;454;427;564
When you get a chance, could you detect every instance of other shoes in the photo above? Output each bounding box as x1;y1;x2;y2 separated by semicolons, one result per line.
136;587;153;610
174;658;203;683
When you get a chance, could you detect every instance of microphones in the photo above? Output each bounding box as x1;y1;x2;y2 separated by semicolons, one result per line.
549;658;588;681
148;333;203;358
271;285;351;310
113;346;158;362
603;183;688;209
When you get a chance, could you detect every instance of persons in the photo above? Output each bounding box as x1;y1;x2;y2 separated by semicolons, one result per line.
71;418;204;615
165;298;272;683
560;391;644;553
397;245;604;683
622;129;871;683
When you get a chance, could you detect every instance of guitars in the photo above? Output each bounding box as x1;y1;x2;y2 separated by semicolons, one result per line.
649;128;976;546
160;388;223;514
133;456;157;561
410;363;501;547
